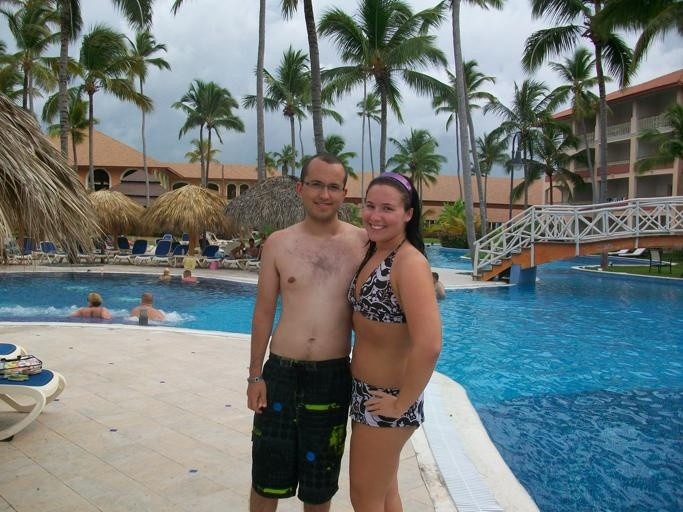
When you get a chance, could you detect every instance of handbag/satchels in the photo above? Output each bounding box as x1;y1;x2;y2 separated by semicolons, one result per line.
1;354;42;376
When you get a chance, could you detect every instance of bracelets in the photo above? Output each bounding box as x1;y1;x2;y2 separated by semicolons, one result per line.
247;375;263;383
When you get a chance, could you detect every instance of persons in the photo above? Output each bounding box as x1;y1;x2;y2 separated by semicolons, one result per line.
70;292;111;320
131;292;165;320
245;153;374;511
244;238;259;260
159;268;172;285
181;269;198;287
229;241;246;259
347;169;444;511
431;271;445;301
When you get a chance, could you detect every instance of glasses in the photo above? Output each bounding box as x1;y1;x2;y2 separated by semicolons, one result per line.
302;180;341;191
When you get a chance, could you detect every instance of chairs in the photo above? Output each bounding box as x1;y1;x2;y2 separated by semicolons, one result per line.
1;340;65;443
647;247;673;275
0;227;260;271
